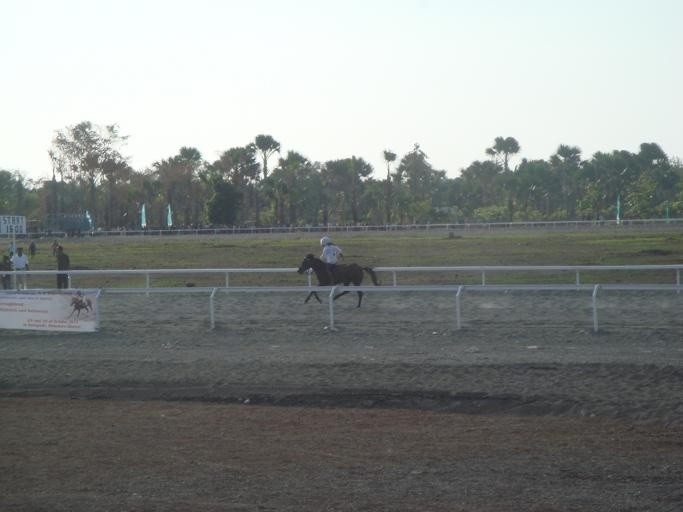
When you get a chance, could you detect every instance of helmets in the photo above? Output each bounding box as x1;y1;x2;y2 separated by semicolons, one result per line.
320;236;332;246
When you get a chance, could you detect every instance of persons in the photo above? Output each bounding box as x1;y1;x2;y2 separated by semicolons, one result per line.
320;237;344;286
56;246;70;288
29;241;37;260
0;242;30;290
51;240;59;256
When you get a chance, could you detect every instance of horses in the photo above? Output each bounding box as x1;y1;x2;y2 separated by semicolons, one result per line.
69;297;93;319
297;253;381;307
